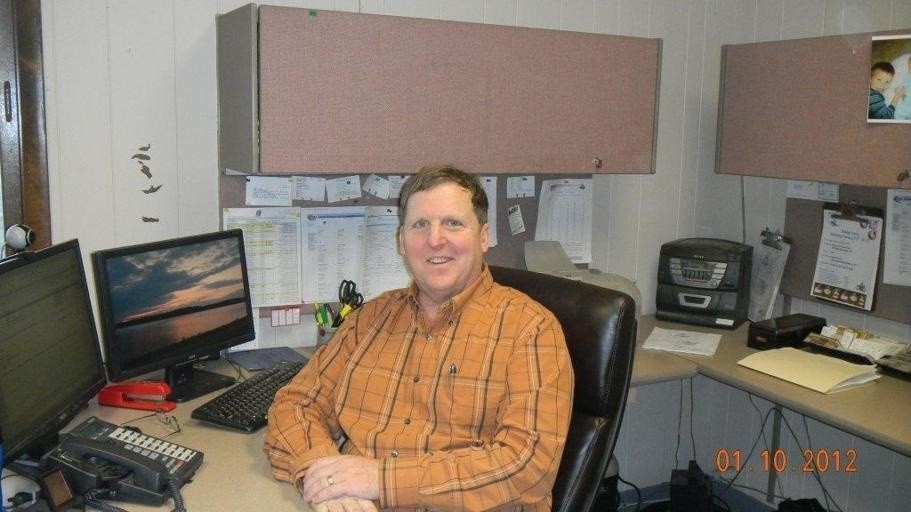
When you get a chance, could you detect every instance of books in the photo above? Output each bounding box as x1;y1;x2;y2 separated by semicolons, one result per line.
735;345;883;396
744;230;793;322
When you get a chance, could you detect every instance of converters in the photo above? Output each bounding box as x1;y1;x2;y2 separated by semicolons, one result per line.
687;457;699;484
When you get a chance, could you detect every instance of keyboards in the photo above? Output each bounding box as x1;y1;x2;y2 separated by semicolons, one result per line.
189;358;304;435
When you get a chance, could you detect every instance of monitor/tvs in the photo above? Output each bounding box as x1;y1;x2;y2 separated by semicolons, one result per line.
0;238;108;483
89;228;256;404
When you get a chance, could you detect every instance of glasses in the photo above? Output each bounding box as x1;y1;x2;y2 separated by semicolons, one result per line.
122;408;181;438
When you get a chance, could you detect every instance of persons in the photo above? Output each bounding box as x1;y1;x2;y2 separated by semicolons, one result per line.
867;59;907;118
262;164;576;512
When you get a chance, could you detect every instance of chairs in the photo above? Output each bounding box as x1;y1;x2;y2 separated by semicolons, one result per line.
485;265;638;511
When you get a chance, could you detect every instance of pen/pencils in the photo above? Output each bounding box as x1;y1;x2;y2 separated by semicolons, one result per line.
315;303;334;330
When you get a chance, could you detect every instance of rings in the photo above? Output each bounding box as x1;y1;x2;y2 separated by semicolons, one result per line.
327;476;333;485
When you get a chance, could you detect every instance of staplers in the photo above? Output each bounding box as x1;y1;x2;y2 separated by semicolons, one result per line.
99;380;176;412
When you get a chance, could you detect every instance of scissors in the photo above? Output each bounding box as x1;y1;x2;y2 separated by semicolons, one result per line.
331;280;364;328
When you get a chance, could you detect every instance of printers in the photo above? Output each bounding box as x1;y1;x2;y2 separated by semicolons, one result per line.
655;237;754;331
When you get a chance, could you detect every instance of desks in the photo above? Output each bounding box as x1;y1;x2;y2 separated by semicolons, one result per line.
1;343;700;511
638;316;910;511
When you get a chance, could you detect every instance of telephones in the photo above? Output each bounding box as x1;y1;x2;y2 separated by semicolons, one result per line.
45;416;204;505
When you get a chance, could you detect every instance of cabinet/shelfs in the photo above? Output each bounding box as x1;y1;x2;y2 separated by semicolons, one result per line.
712;27;911;191
217;3;664;174
